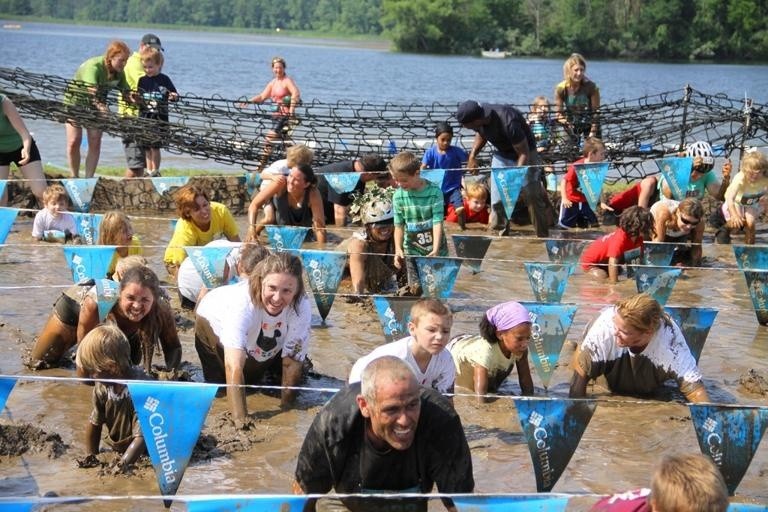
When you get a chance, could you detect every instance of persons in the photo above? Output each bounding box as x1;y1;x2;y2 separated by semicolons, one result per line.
446;300;535;405
586;453;730;512
349;298;459;407
555;137;768;284
137;46;180;177
26;183;311;474
239;57;301;173
118;34;164;178
0;93;48;207
293;355;475;512
248;96;551;296
568;293;711;404
63;41;139;179
555;53;601;163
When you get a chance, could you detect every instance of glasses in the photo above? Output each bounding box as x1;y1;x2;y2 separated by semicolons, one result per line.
680;214;701;226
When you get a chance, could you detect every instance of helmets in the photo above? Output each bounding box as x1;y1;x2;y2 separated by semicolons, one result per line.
359;198;394;225
685;141;715;173
283;96;302;106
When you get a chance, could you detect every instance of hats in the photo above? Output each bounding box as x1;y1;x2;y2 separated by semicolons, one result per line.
457;99;484;124
142;33;164;52
